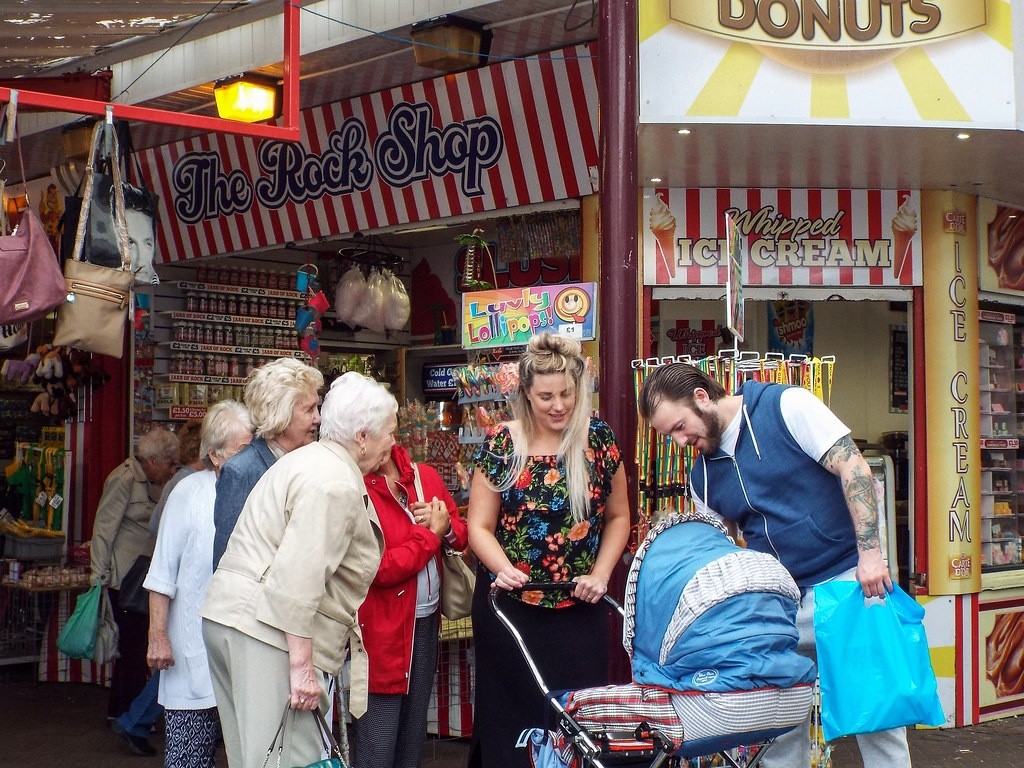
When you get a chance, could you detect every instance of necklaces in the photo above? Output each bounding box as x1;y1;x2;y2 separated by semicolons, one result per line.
398;490;408;504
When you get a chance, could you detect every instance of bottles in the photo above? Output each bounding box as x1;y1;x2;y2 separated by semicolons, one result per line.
994;422;1008;435
9;559;24;584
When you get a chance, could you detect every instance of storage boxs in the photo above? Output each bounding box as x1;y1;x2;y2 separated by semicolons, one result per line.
3;536;64;559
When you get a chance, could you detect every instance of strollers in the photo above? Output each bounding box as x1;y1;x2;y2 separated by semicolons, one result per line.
489;512;817;768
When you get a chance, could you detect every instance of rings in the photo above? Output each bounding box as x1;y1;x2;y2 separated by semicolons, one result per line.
299;698;305;703
587;595;591;600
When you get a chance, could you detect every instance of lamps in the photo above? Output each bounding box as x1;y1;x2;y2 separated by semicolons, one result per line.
61;119;95;163
214;72;282;124
409;14;493;72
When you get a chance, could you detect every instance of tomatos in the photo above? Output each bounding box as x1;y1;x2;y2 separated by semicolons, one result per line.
584;356;595;380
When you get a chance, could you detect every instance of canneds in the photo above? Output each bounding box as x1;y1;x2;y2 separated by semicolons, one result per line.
168;263;305;378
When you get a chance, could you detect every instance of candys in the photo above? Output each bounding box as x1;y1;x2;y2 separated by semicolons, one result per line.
394;404;437;458
453;363;522;489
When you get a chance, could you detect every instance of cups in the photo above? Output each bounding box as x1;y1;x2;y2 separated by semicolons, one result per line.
442;326;452;344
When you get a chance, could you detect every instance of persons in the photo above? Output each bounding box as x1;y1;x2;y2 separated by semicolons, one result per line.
201;373;397;768
212;357;324;575
92;428;181;756
147;400;256;767
467;331;631;767
638;361;915;768
351;441;446;768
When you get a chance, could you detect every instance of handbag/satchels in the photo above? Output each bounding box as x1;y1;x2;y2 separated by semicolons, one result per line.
260;699;350;768
410;459;474;621
55;581;121;664
812;580;946;741
0;105;161;358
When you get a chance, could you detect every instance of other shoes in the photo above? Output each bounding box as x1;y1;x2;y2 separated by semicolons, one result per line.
111;722;157;756
108;717;157;732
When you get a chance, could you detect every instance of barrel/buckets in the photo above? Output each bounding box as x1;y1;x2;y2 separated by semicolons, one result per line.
294;305;316;333
301;335;320;358
296;263;318;292
308;286;330;314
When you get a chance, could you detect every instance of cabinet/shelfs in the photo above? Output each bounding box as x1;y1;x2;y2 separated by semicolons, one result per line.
974;310;1024;573
154;279;309;409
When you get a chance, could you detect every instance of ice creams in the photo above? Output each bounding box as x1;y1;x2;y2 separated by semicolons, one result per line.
891;194;917;278
650;192;678;278
